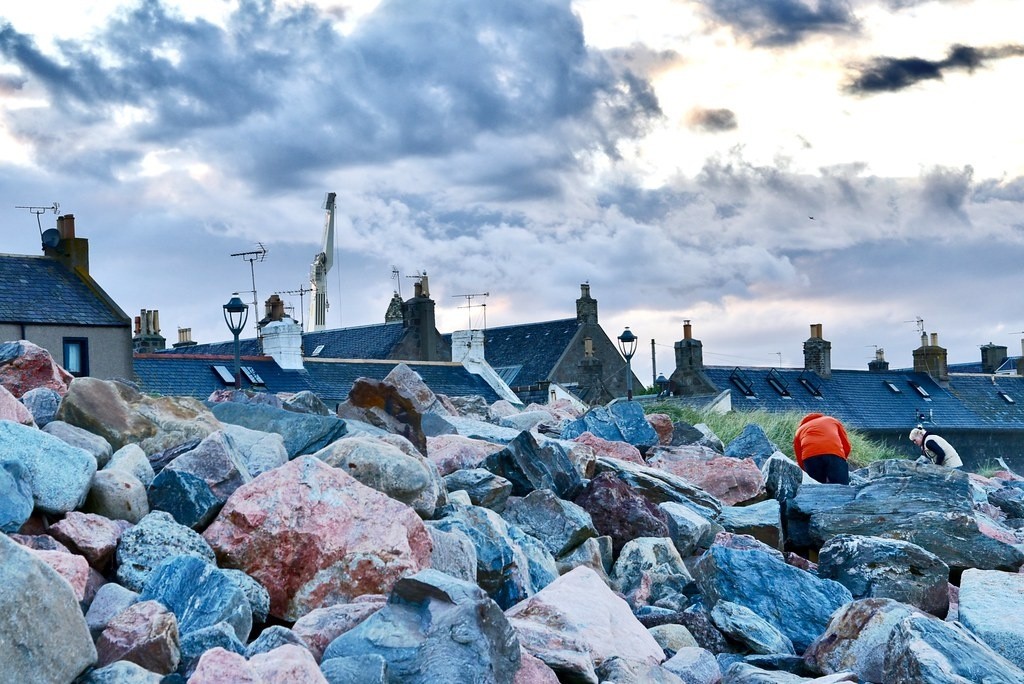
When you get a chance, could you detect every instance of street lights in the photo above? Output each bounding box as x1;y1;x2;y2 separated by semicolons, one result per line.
616;326;639;401
221;291;249;391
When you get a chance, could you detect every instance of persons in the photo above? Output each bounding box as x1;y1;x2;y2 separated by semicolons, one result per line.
794;413;851;485
909;425;963;470
